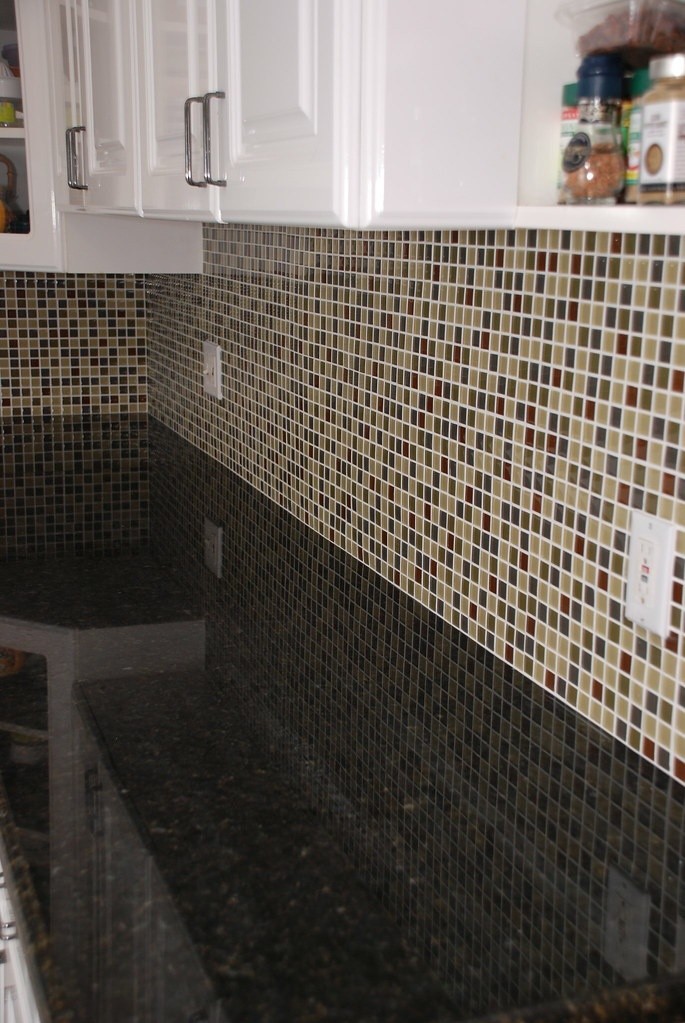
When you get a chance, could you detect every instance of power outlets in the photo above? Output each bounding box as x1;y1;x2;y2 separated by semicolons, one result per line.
202;341;222;399
202;517;223;579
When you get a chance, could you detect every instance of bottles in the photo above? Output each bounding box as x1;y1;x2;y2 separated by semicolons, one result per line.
559;52;684;205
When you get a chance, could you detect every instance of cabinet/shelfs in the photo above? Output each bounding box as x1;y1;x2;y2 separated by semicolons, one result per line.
0;0;524;275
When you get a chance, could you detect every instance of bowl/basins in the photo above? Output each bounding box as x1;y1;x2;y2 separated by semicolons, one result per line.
556;0;685;69
